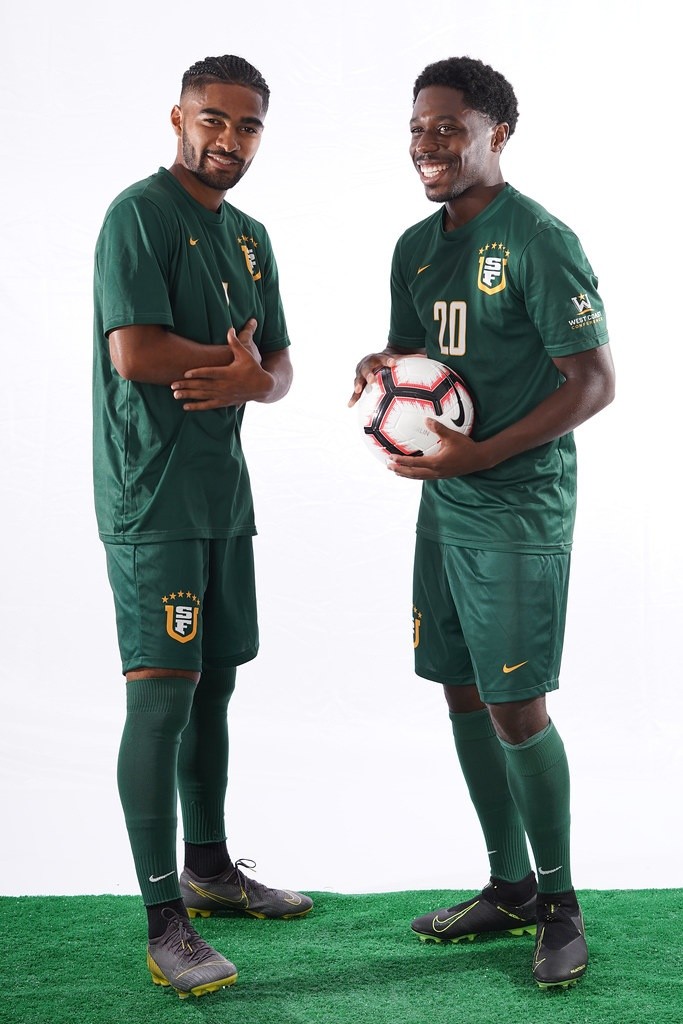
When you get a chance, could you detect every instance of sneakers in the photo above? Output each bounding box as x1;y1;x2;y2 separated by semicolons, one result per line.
534;897;591;990
411;884;543;946
146;917;237;999
179;865;315;922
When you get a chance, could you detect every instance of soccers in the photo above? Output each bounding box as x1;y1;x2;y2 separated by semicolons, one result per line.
363;355;474;463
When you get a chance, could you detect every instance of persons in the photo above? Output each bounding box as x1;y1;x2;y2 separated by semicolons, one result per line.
92;55;315;994
347;55;617;990
